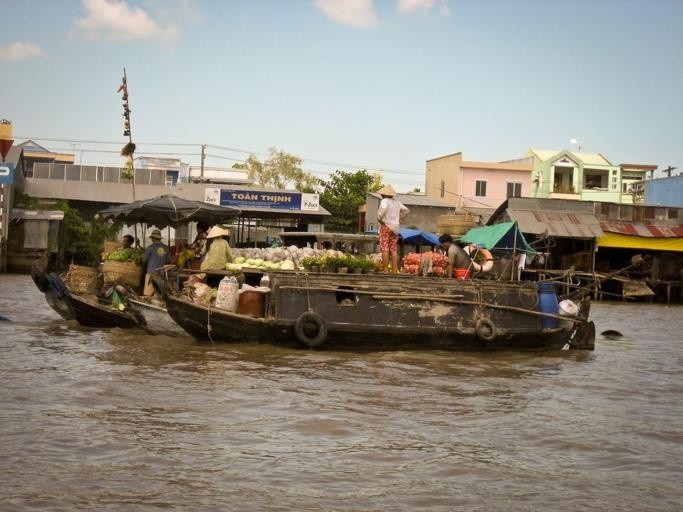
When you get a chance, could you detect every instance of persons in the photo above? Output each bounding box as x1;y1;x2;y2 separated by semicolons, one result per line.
437;233;474;279
374;184;411;273
116;222;233;298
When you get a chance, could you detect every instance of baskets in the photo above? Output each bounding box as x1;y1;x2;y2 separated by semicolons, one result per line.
65;259;143;293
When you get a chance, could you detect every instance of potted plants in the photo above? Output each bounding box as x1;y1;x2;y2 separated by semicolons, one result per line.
299;251;374;274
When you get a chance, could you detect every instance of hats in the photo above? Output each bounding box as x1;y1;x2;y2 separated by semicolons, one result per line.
149;230;164;239
206;226;227;238
377;186;396;196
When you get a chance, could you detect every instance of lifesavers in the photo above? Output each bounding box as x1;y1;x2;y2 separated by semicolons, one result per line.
462;244;494;273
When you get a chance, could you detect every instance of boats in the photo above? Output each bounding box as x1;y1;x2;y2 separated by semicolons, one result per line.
29;254;146;328
127;294;193;339
150;219;595;352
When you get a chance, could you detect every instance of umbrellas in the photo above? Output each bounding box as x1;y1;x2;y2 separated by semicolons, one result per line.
93;192;240;245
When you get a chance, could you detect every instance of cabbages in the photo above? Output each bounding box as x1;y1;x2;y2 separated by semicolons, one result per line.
225;257;294;271
